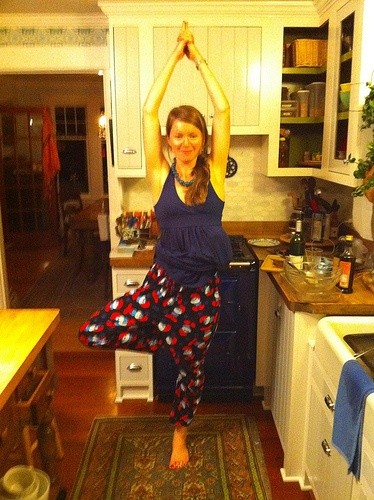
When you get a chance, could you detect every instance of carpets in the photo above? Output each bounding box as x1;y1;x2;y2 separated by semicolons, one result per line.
66;410;273;500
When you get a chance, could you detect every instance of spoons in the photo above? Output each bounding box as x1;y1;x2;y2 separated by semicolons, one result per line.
314;188;321;199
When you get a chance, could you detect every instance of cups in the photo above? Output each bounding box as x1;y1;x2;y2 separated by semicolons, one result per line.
304;248;323;267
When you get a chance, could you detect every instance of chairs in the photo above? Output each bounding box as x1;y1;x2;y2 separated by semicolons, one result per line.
54;192;110;278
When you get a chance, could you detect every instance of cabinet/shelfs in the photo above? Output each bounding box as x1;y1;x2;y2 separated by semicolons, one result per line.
109;263;274;403
300;349;374;500
92;0;374;189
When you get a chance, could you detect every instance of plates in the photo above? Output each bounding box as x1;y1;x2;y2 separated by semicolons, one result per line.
261;254;285;272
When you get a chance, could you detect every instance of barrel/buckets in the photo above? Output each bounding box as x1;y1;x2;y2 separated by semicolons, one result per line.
0;464;50;500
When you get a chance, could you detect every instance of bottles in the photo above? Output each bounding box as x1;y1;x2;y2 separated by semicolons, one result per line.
332;234;356;294
289;219;306;270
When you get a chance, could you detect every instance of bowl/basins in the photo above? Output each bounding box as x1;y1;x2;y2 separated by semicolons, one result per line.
285;256;343;293
339;83;350;109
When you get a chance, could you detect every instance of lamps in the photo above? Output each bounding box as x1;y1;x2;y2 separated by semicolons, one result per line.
95;106;106;129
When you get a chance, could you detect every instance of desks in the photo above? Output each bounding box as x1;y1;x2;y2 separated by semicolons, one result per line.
71;198;108;262
0;302;67;500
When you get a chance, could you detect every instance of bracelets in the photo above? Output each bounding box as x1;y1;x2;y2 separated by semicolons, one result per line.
196;60;206;70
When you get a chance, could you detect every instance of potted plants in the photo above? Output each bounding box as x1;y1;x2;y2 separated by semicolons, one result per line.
342;75;374;204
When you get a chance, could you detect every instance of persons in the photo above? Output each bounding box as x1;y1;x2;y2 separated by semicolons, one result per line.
79;19;233;470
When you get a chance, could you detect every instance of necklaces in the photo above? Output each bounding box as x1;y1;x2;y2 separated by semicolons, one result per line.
171;162;194;187
